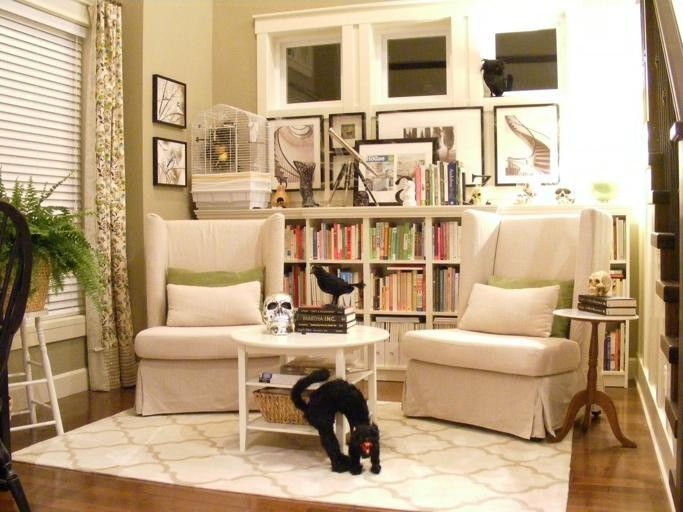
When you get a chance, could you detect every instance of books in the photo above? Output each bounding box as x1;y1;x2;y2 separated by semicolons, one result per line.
280;357;337;377
283;224;306;307
432;221;462;329
416;161;464;205
369;221;426;343
309;222;362;308
577;217;638;371
294;306;362;333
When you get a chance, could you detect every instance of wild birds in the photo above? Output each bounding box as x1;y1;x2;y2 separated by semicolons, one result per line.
310;263;366;305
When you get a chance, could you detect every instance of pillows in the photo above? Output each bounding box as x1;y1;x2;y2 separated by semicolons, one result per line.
166;281;264;325
488;277;575;337
458;282;560;338
166;265;265;311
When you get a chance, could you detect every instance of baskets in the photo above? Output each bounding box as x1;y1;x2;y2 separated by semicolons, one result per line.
252;387;313;425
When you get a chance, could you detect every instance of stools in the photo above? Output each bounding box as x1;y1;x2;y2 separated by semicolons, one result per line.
9;308;65;435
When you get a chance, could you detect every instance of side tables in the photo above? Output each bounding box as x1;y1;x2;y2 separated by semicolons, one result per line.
546;302;642;449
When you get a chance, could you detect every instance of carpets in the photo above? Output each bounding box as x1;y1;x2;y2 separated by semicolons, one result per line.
12;400;574;512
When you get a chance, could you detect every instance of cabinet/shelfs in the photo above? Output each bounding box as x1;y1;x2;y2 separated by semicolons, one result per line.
196;207;630;390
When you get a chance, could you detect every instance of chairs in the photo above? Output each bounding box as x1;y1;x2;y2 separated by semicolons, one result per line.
0;201;31;512
133;212;286;416
402;208;614;440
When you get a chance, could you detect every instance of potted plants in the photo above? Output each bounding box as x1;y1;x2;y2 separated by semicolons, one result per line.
0;165;109;315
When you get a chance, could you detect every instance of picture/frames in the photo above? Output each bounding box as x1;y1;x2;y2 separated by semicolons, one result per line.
375;106;485;187
329;152;355;190
152;74;187;129
494;103;559;186
266;115;324;192
328;112;366;151
152;137;188;187
353;138;439;207
